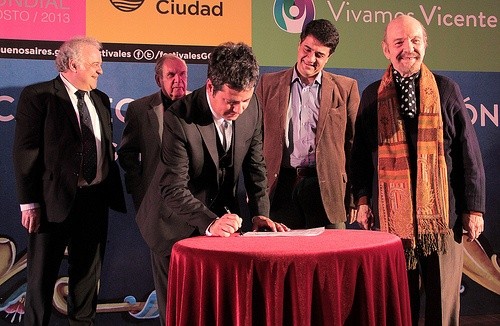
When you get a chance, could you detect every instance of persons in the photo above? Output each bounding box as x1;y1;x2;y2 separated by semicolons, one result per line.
351;15;486;326
250;19;361;230
133;42;292;326
13;37;128;326
116;54;197;216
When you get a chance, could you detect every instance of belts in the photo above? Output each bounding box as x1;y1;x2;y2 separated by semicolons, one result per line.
280;166;316;178
75;184;104;194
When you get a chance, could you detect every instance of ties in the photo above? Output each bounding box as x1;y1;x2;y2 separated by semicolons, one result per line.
222;120;231;152
74;88;98;185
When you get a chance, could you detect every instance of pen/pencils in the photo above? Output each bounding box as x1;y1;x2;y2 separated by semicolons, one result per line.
224;206;245;234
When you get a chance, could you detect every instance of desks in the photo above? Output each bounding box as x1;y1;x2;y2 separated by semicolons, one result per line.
163;228;413;326
